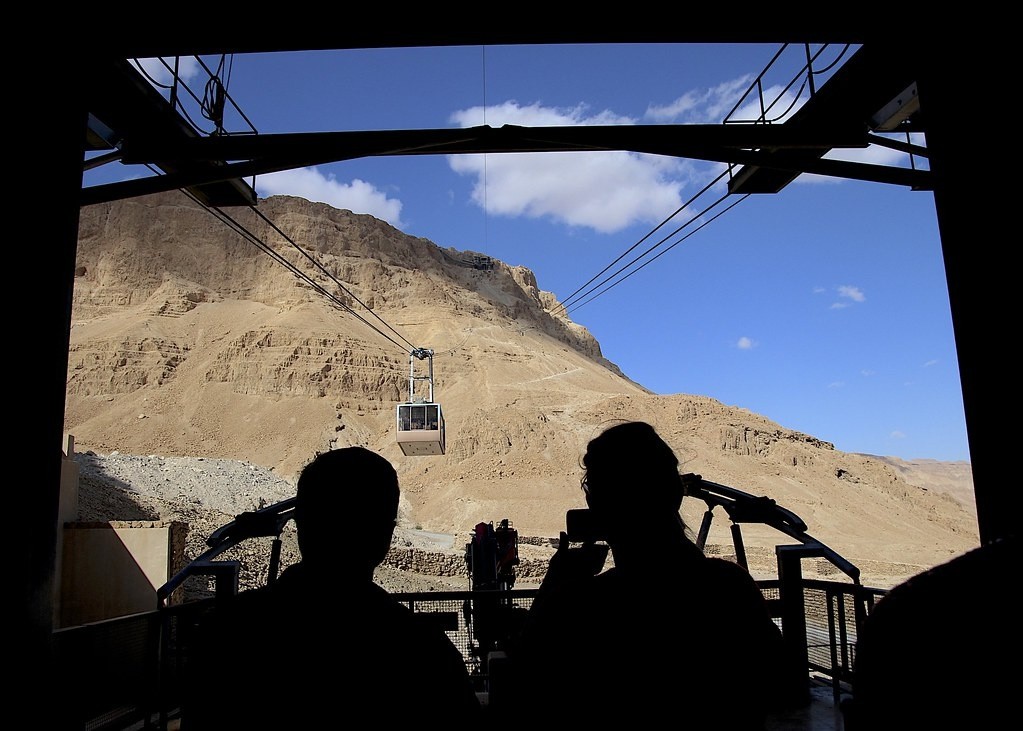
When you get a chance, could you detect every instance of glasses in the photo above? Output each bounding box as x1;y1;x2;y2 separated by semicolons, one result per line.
581;481;590;495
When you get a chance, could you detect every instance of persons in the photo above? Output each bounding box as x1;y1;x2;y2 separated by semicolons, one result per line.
492;421;806;731
464;519;518;591
179;447;485;731
851;530;1023;731
